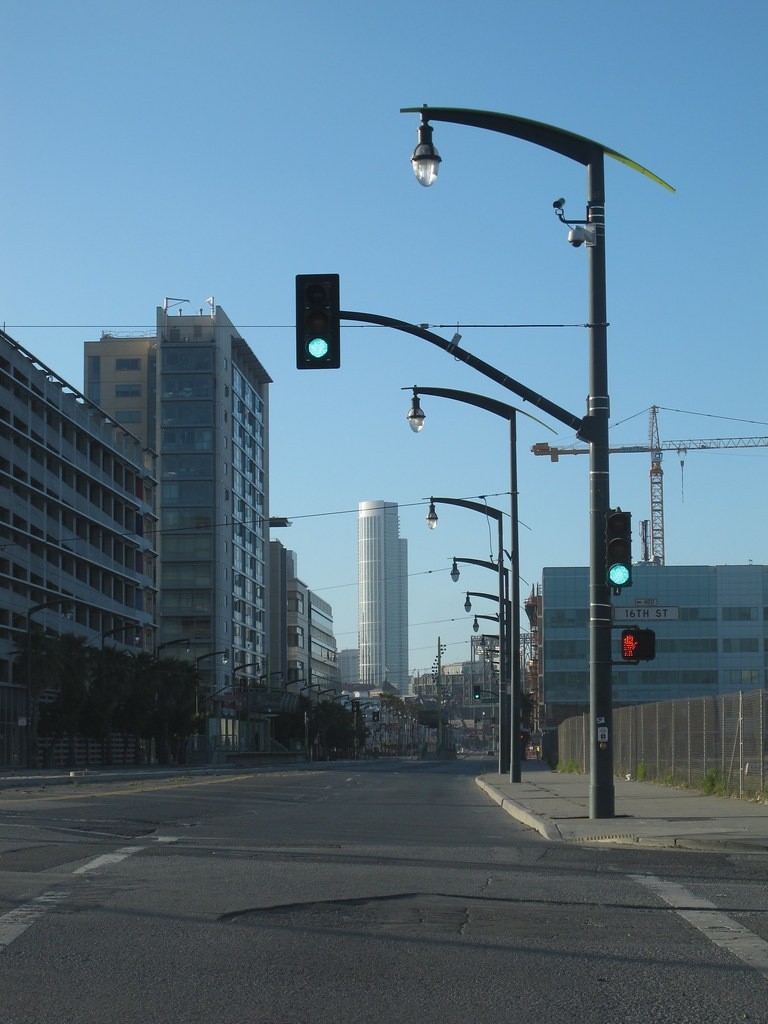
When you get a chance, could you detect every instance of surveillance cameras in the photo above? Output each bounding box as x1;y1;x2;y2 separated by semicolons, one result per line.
552;198;565;209
568;231;585;248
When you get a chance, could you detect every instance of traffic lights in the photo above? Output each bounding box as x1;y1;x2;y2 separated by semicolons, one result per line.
474;685;480;699
622;629;656;661
295;274;341;369
606;511;633;587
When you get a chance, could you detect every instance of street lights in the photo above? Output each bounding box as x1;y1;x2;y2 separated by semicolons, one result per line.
101;626;142;648
25;600;78;769
259;671;284;687
401;385;522;784
332;695;350;703
153;638;191;659
364;704;379;725
400;103;615;819
285;679;308;691
344;698;360;708
232;662;261;751
299;684;320;695
317;689;337;702
447;556;511;769
421;497;508;775
195;651;229;714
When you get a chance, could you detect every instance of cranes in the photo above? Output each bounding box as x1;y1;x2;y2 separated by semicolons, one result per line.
529;405;767;565
